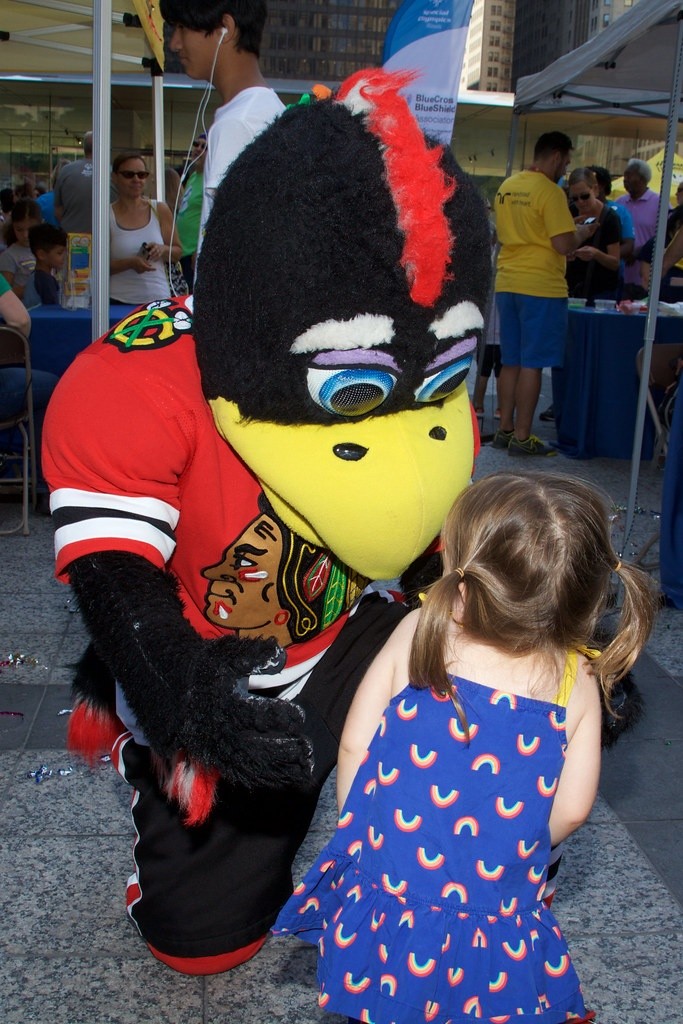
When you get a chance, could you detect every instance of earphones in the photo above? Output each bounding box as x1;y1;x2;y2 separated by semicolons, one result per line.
218;27;228;45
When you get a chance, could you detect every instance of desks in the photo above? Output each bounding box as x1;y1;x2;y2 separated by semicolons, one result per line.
551;302;683;460
0;303;141;495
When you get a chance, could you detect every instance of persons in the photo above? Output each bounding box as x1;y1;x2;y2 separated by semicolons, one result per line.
474;129;683;457
1;130;209;515
272;472;654;1022
158;0;288;296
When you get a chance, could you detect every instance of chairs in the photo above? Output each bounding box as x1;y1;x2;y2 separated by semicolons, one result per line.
0;325;37;536
635;344;683;569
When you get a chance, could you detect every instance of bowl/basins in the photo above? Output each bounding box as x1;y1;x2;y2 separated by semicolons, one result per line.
621;303;641;315
595;299;616;311
568;298;587;309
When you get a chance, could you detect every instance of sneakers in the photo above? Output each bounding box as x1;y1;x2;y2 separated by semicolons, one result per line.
489;427;516;449
508;434;557;458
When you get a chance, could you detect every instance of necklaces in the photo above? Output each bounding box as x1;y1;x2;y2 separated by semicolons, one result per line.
527;165;553;181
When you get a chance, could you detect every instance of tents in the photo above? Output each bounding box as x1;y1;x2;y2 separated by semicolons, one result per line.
476;1;683;549
0;0;166;349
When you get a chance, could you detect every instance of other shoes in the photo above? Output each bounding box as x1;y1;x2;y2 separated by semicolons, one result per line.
35;494;50;514
494;408;500;419
474;407;484;417
539;406;555;421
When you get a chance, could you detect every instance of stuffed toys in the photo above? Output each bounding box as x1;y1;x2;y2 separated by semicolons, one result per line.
41;66;644;976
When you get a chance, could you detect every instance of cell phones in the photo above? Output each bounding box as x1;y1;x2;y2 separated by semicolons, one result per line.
584;217;596;224
137;242;152;261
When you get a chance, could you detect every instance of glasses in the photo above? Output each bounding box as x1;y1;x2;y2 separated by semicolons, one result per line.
118;171;149;179
193;141;207;151
569;189;593;202
678;188;683;193
